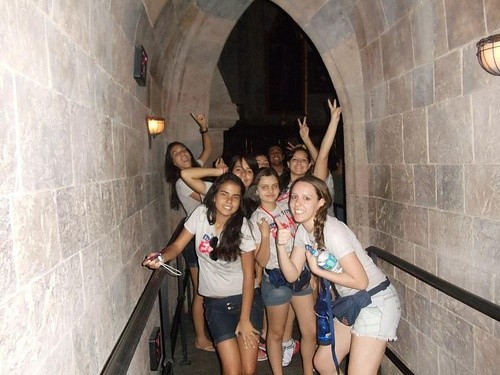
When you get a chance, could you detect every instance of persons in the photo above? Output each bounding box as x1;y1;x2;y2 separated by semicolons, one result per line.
274;176;402;375
165;99;344;367
143;172;263;375
249;167;316;375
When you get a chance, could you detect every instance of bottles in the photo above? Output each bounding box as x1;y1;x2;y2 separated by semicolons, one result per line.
314;294;332;345
311;249;344;274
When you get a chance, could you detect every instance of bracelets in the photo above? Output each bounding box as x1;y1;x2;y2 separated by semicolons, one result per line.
200;127;209;134
221;167;226;174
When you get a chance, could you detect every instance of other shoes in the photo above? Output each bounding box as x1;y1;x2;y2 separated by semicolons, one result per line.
282;339;300;367
255;338;269;362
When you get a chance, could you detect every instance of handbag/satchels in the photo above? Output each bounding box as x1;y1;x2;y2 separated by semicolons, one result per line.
265;267;311;292
332;275;391;327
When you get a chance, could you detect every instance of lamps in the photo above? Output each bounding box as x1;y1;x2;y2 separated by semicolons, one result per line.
475;33;500;76
147;114;166;138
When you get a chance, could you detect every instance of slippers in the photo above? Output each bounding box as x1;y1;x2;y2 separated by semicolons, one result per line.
195;340;217;352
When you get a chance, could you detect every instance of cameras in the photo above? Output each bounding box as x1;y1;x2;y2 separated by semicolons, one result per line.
148;254;162;265
